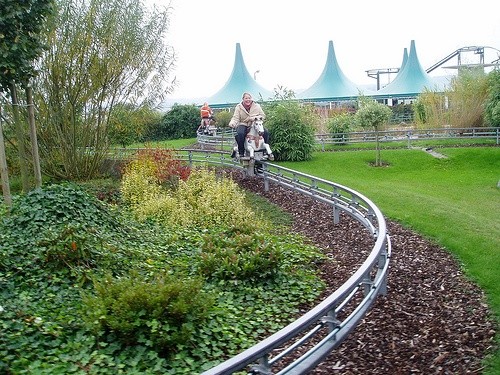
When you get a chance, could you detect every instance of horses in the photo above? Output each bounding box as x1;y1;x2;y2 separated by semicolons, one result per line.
230;117;275;163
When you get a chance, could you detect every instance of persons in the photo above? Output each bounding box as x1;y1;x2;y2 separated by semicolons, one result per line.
201;103;214;127
228;92;271;156
